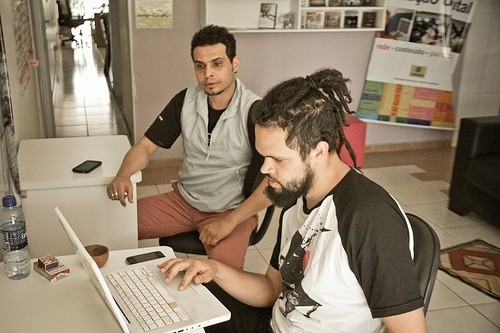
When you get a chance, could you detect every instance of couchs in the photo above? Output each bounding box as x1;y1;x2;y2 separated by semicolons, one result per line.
448;116;500;231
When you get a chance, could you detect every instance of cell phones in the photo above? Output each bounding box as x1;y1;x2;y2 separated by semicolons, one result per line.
126;251;166;265
72;160;103;174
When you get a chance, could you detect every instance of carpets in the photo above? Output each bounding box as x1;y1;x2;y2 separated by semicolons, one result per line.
439;239;500;300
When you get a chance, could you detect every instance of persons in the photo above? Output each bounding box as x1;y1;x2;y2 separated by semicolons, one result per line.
157;68;427;333
105;24;275;272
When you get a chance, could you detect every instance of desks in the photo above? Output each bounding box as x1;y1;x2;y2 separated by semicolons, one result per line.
0;246;206;333
16;135;142;259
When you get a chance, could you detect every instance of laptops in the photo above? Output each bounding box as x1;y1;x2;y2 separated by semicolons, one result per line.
54;206;232;333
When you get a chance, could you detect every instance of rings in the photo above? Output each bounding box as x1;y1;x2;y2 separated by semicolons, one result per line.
111;193;114;197
115;192;118;196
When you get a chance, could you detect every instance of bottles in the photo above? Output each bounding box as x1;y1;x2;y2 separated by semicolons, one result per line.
0;195;31;280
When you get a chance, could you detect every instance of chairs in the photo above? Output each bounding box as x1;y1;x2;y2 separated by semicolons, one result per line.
159;160;274;255
56;0;85;47
405;211;441;320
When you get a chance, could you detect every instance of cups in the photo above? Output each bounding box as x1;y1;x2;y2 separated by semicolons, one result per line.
84;244;109;268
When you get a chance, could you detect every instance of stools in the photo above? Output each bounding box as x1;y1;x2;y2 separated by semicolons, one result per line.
338;113;367;168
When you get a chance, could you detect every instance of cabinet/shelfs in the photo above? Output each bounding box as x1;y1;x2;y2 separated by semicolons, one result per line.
201;0;387;34
